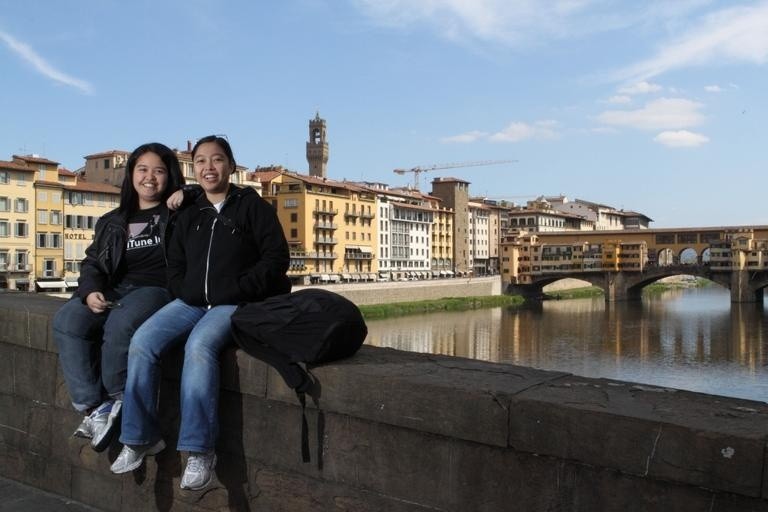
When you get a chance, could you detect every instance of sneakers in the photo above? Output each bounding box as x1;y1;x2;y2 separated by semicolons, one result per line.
83;400;123;453
110;438;166;475
180;451;217;492
73;413;94;440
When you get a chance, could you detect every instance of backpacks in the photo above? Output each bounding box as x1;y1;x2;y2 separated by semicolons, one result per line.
231;288;369;464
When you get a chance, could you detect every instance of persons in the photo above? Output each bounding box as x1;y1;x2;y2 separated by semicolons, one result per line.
50;141;203;453
108;133;293;491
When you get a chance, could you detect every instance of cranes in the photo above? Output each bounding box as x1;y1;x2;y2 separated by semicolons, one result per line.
393;159;519;190
